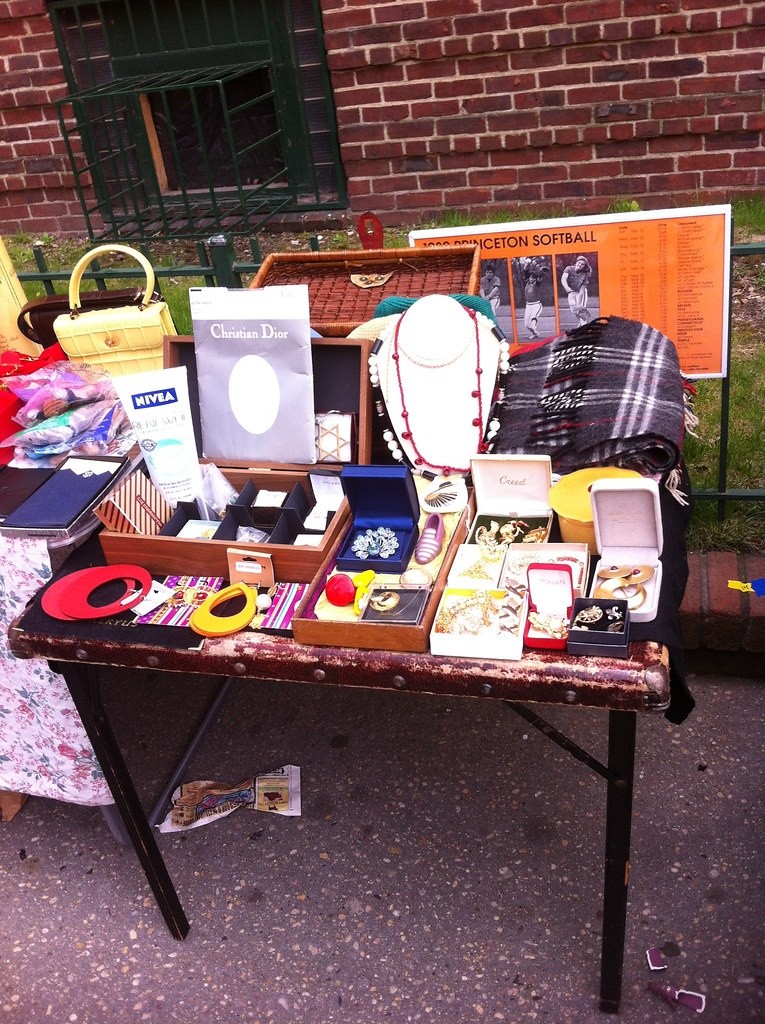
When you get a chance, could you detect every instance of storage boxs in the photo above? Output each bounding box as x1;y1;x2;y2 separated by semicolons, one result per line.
97;333;375;585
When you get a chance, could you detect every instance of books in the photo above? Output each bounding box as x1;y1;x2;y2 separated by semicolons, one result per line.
1;457;122;529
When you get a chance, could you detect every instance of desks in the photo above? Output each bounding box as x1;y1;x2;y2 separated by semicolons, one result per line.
8;519;671;1012
0;437;146;577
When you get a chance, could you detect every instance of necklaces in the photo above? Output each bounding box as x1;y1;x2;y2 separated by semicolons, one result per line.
385;308;489;476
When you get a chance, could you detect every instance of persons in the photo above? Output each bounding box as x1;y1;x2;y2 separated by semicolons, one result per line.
479;255;593;339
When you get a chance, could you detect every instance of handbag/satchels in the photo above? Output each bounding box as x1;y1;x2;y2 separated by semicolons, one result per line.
51;245;178;380
